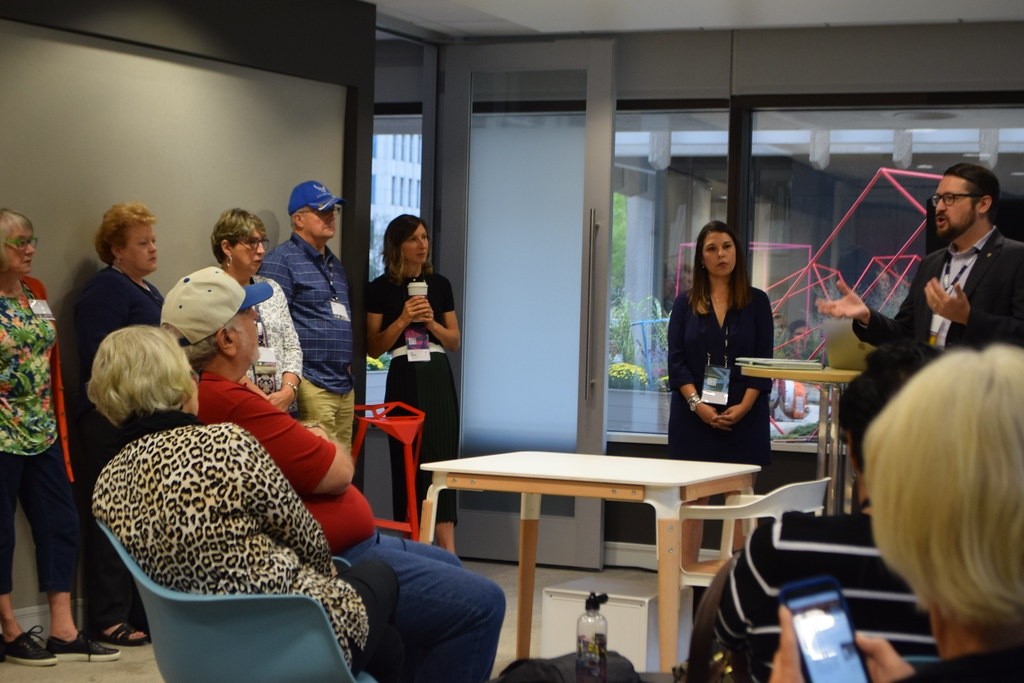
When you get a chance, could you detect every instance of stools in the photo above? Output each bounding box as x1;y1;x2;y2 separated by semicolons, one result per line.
350;402;427;540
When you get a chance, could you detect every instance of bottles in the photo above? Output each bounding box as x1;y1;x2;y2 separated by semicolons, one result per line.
573;589;611;683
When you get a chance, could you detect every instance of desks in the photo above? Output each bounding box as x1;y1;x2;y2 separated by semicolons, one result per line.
418;451;763;675
742;365;865;515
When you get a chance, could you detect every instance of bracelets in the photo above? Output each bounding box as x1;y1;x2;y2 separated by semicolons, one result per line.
283;383;298;404
688;394;700;407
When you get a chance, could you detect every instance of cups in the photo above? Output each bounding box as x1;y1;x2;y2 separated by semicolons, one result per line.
408;281;430;325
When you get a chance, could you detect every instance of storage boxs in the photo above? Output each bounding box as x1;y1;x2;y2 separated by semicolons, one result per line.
542;568;697;675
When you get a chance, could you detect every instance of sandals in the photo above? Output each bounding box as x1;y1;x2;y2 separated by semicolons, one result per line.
85;622;147;644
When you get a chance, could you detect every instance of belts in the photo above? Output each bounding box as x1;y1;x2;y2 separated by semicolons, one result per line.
391;342;445;358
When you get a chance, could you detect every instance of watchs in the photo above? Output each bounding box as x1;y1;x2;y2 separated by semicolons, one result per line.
690;401;702;413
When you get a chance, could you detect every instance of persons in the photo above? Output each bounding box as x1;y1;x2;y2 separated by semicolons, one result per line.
768;343;1024;683
815;164;1024;355
0;208;119;670
788;320;811;360
214;207;303;421
666;221;774;498
686;344;942;683
87;325;399;676
257;180;355;464
161;267;505;683
367;215;460;554
72;201;165;644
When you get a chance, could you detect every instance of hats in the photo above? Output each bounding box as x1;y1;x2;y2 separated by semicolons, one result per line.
160;266;272;346
289;181;345;214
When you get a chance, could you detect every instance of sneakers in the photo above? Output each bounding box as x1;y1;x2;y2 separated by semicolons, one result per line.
1;626;57;666
46;631;120;661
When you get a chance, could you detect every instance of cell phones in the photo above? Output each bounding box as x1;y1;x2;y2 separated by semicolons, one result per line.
778;576;872;683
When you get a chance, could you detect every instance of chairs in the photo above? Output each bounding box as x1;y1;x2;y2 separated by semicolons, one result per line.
96;516;384;683
683;473;834;587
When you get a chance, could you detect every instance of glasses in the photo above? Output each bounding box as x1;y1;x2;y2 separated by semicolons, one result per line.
297;207;340;217
932;193;986;206
226;237;270;251
5;237;38;250
